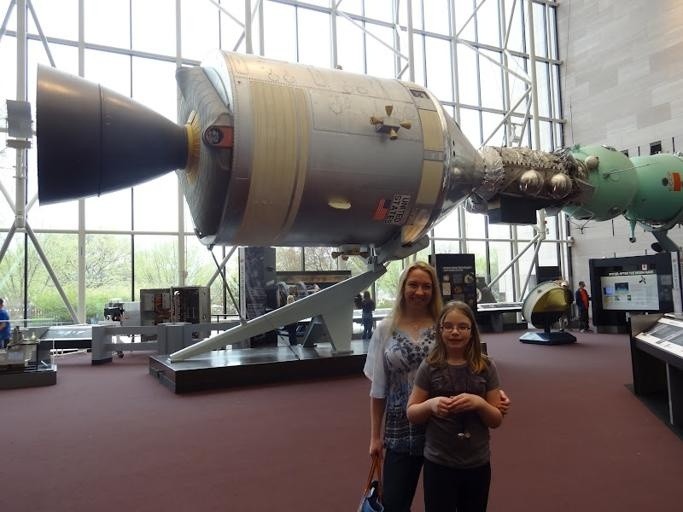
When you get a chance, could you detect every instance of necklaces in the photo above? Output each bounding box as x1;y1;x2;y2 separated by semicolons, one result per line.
401;313;427;331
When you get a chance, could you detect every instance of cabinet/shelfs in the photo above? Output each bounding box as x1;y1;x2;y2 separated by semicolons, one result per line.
139;286;211;343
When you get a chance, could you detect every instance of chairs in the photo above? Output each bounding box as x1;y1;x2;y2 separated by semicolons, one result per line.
0;325;33;372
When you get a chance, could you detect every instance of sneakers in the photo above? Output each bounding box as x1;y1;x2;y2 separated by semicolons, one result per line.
559;327;592;332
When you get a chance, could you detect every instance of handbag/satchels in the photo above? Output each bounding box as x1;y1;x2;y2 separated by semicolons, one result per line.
356;454;385;511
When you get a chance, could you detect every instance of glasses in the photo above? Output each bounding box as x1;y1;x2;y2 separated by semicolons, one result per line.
440;326;471;332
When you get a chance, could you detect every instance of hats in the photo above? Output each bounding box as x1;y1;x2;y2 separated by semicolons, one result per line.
561;282;569;287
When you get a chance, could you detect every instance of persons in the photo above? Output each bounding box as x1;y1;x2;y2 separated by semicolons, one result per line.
558;279;574;333
362;260;512;512
0;298;11;349
361;291;375;339
575;281;593;333
285;295;298;345
406;300;504;512
